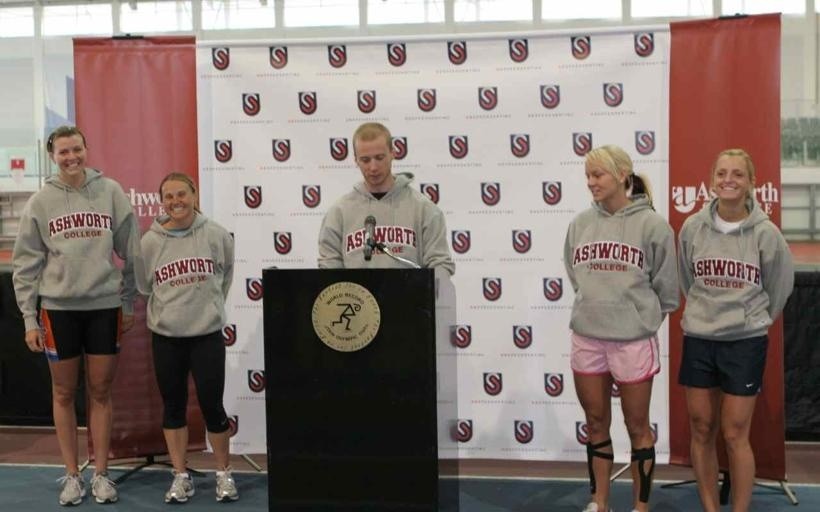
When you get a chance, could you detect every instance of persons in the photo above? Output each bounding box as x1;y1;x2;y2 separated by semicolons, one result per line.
318;123;457;279
677;148;794;512
565;145;680;512
137;172;239;505
12;125;136;505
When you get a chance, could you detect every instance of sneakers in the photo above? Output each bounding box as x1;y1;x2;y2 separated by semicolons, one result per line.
163;470;196;503
89;471;119;504
54;471;87;507
214;464;239;502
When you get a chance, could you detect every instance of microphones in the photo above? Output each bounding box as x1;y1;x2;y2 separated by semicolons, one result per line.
361;215;379;260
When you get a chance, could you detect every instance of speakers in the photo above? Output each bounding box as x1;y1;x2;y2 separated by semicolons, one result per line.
783;264;820;443
0;267;88;428
